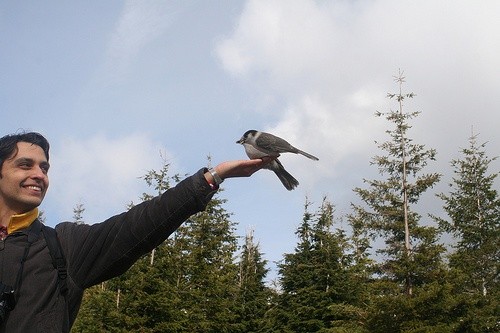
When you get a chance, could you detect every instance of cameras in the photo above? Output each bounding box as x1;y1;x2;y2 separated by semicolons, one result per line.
0;287;17;323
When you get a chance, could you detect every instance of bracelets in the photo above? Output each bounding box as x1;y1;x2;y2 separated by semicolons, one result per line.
208;167;225;186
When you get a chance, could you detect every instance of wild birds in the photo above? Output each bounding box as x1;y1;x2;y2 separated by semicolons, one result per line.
241;130;319;161
236;136;299;191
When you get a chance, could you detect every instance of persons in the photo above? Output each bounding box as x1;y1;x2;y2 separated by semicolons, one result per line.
0;132;280;333
